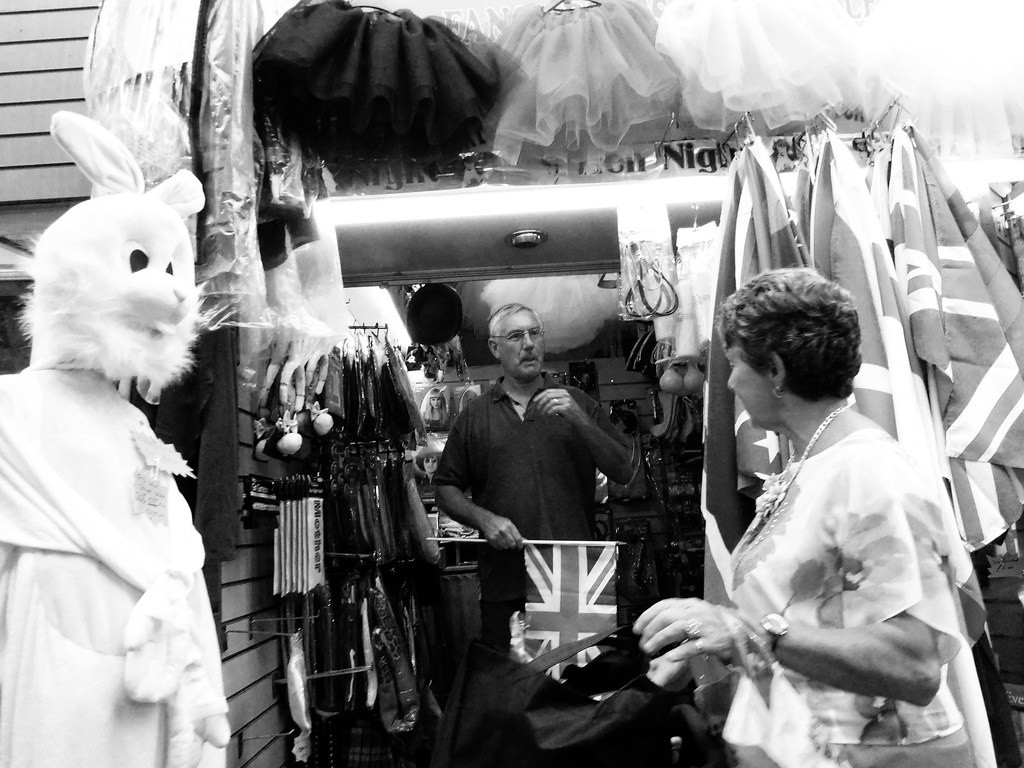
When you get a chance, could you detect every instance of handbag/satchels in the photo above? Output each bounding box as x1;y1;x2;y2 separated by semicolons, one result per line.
429;623;739;768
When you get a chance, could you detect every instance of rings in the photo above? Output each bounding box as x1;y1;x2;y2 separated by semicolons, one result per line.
685;620;703;637
695;640;703;655
554;399;559;405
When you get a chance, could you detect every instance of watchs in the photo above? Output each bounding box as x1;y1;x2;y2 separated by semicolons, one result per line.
759;613;788;651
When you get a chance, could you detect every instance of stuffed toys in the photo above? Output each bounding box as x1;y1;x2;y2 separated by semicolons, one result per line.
0;110;231;768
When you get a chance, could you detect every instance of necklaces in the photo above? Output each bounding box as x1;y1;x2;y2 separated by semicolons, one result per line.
734;404;846;570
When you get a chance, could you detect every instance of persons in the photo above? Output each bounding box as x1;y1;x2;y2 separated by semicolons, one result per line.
417;446;441;484
431;303;633;654
421;388;448;421
632;268;996;768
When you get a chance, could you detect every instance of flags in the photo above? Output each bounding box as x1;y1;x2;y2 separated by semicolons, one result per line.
525;543;617;681
701;119;1024;647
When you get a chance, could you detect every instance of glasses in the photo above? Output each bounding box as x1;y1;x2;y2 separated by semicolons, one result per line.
490;329;545;339
